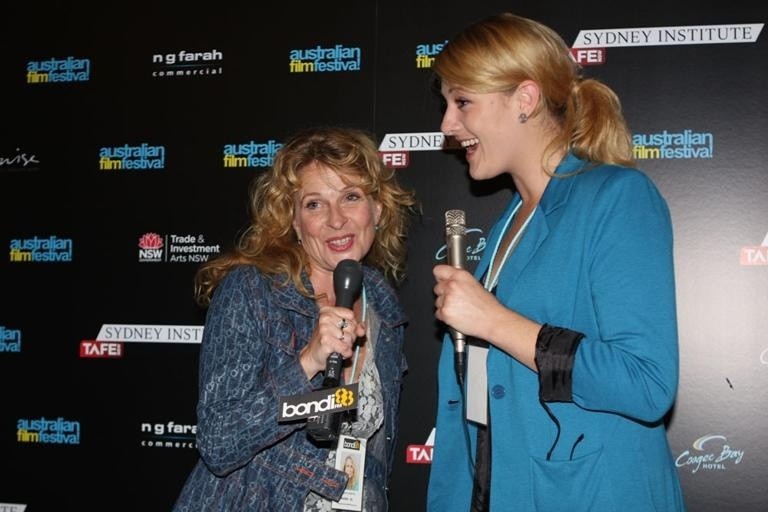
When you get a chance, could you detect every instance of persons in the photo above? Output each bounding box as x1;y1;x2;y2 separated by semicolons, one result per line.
170;127;424;511
343;456;359;492
426;12;687;511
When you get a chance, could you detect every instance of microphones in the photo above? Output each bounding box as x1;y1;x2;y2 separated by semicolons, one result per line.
443;208;466;385
324;258;364;382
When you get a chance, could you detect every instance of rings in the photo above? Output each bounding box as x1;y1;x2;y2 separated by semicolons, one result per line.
338;330;346;341
340;319;346;328
